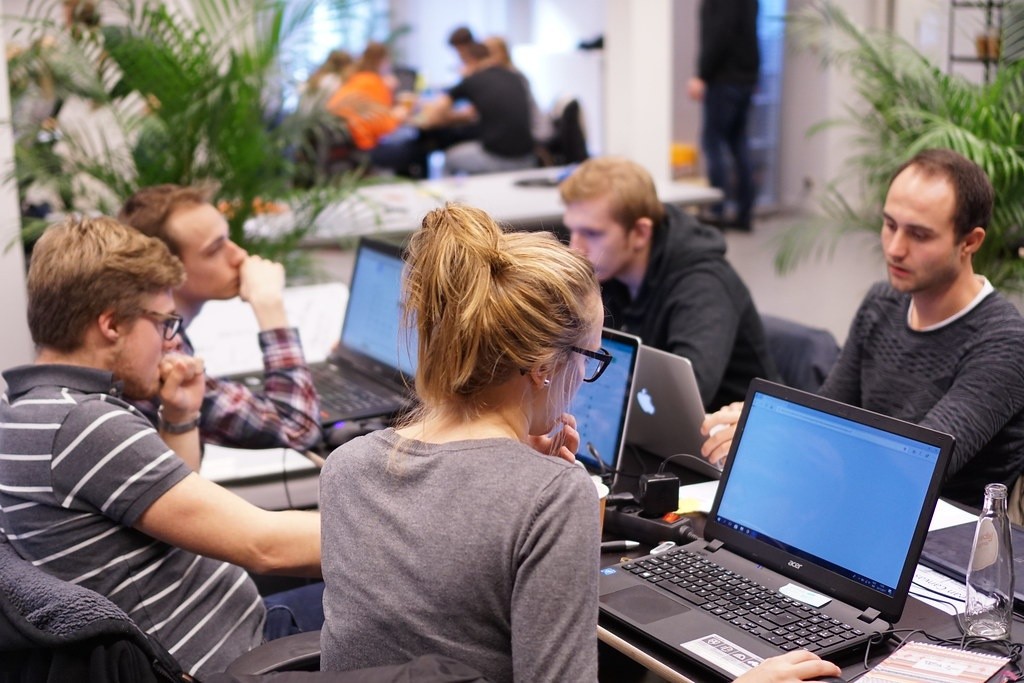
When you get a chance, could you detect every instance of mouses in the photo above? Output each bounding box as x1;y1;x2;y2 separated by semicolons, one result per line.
804;675;846;683
321;418;384;449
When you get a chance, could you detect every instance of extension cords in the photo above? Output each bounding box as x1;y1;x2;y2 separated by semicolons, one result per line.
604;503;692;547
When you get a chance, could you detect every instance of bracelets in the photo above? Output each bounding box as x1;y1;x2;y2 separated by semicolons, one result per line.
157;404;201;434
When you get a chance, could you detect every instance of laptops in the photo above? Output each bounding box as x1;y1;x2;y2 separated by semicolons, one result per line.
623;345;726;480
216;236;420;429
918;520;1024;614
599;377;955;683
543;326;642;491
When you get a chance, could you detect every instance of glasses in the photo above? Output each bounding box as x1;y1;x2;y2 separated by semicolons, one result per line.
138;307;183;340
516;346;613;383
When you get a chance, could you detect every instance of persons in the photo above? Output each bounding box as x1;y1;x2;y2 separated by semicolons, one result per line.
690;0;759;234
318;202;841;683
293;43;416;179
560;157;787;415
700;149;1024;517
412;27;554;176
116;184;321;453
0;214;323;679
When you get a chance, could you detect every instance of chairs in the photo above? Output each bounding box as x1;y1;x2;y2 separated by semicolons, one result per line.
0;544;320;683
764;315;841;390
536;100;588;163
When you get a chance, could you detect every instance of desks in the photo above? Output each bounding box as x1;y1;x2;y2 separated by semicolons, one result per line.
38;161;725;250
392;122;483;175
296;453;1024;683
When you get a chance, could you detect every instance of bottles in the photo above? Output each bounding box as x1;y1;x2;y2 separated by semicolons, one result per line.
964;484;1014;640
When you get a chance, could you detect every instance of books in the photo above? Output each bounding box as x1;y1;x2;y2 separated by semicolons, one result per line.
853;641;1011;683
